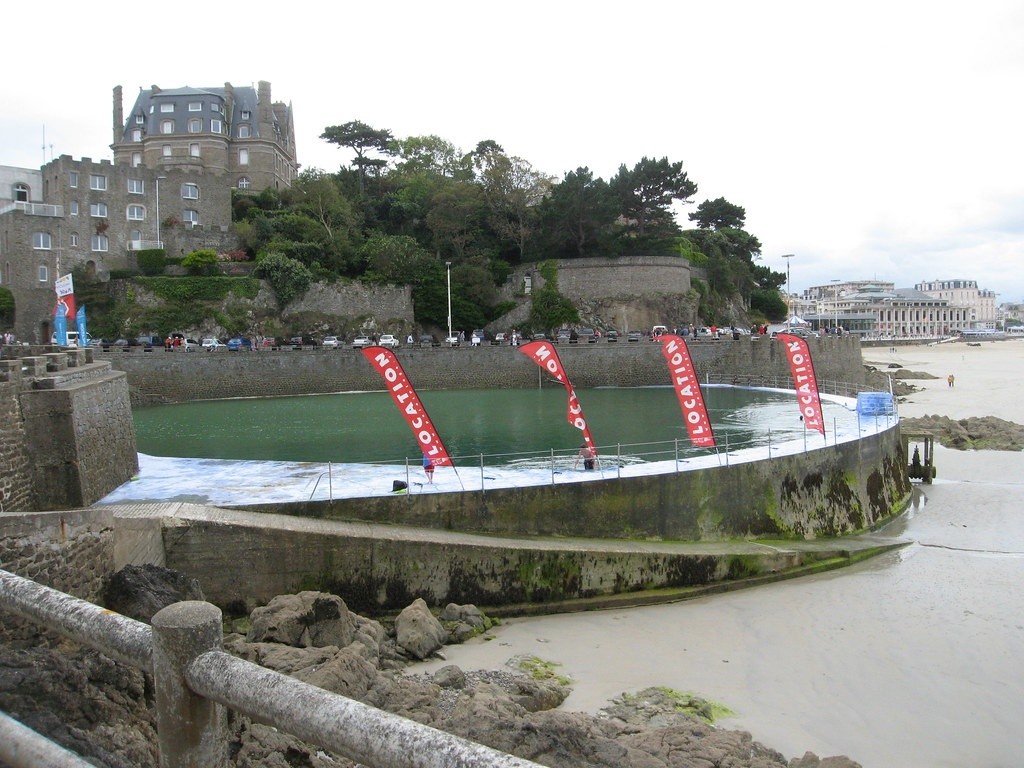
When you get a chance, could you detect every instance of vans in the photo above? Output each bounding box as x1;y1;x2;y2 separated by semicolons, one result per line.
51;331;92;346
653;326;668;334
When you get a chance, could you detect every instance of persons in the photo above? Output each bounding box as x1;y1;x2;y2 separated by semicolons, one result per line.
824;324;843;336
198;336;204;347
511;329;519;346
593;329;601;343
210;336;218;352
0;330;17;346
750;324;770;335
421;450;435;484
654;327;669;343
371;332;378;347
408;333;414;348
948;374;954;388
706;324;717;338
890;347;897;353
461;331;465;344
729;322;735;338
249;335;269;350
672;323;700;341
643;327;650;343
165;337;185;352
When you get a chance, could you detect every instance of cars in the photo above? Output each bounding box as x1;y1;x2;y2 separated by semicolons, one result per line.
473;329;487;340
183;339;200;352
701;327;752;336
608;330;618;342
533;331;546;339
352;336;376;348
228;338;250;351
86;338;137;345
264;336;285;347
579;329;596;339
628;331;638;341
495;332;508;341
420;335;440;346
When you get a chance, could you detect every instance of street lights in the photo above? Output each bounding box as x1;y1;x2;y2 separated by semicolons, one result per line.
446;262;452;336
153;176;168;249
782;255;794;334
831;279;841;334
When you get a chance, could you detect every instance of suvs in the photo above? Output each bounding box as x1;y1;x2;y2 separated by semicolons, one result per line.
323;336;346;347
379;335;399;348
137;336;165;348
289;336;317;345
203;339;227;350
554;330;577;343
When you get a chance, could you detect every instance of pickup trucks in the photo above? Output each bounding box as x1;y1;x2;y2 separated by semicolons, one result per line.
445;331;461;346
